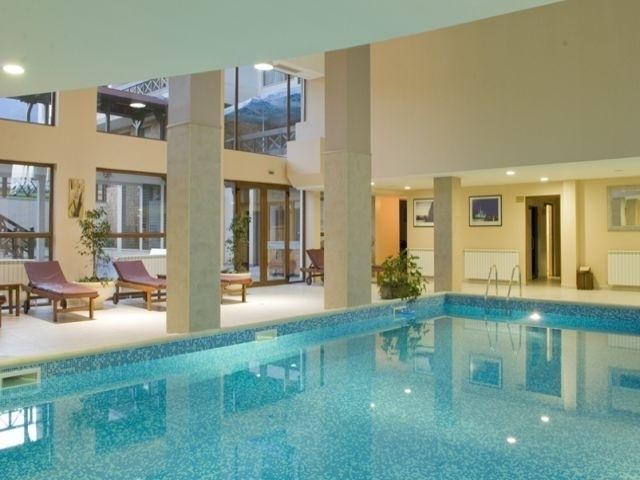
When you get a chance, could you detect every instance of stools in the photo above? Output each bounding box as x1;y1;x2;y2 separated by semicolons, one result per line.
0;281;20;317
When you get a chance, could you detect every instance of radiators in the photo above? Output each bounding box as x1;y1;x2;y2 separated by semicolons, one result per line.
408;247;520;283
0;259;36;308
605;249;640;289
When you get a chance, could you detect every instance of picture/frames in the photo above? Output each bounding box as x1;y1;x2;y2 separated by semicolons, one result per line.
467;194;503;227
412;198;434;228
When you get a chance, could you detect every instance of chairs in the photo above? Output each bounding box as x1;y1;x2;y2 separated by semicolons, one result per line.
301;249;324;285
24;259;99;322
112;260;166;310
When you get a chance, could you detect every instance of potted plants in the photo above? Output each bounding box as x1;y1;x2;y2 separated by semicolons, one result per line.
70;204;119;310
378;249;429;303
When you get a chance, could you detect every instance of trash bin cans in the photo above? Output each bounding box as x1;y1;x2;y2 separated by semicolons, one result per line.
577;266;593;290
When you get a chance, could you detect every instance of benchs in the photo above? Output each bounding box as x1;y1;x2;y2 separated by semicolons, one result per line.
158;272;253;305
